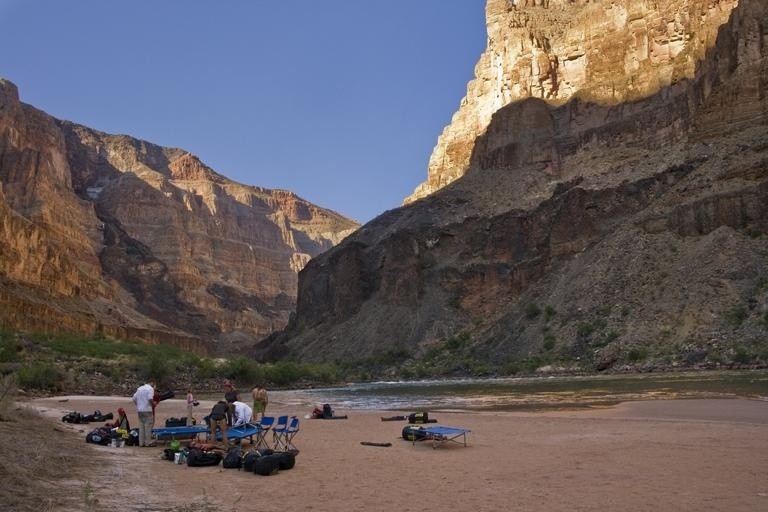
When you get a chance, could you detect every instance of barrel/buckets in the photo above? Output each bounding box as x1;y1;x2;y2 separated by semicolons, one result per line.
402;426;427;441
174;453;182;465
408;412;429;424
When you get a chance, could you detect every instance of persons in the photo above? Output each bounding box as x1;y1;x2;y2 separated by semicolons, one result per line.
227;401;256;445
130;378;158;446
186;387;197;427
223;378;269;427
151;383;161;428
208;400;233;451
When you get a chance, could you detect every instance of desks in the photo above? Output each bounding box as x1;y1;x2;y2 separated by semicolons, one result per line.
409;426;472;449
209;421;263;442
152;426;211;442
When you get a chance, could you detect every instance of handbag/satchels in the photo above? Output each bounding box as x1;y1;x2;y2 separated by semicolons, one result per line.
86;424;139;447
164;449;295;475
62;410;113;424
165;417;196;428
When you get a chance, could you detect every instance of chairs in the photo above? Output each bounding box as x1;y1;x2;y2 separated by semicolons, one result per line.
254;416;299;453
